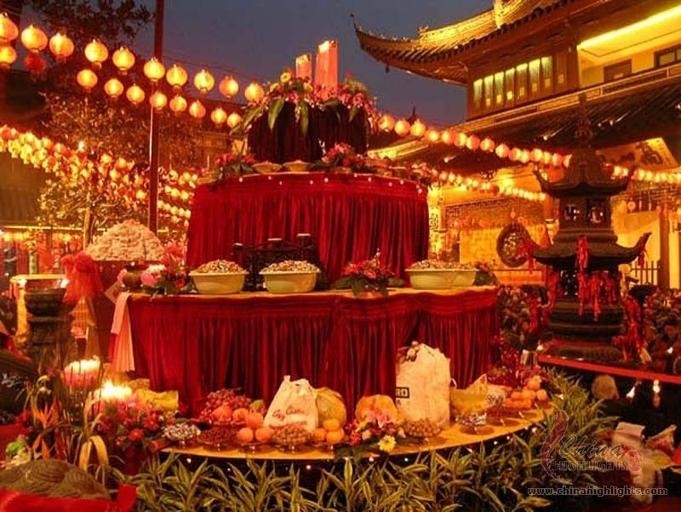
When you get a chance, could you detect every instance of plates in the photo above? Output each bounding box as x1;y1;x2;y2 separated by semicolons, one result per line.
252;162;315;174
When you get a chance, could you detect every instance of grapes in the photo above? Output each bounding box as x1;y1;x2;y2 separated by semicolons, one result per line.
200;388;253;418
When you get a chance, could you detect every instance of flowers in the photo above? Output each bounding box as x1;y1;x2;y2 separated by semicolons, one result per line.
211;150;256;169
116;243;192;296
227;76;378;146
476;257;499;284
90;394;178;451
318;143;364;170
332;251;394;294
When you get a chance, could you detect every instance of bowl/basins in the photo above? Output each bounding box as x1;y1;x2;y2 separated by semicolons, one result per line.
259;271;322;293
188;270;250;294
405;268;479;290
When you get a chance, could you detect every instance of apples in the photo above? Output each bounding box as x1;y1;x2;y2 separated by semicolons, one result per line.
235;411;270;444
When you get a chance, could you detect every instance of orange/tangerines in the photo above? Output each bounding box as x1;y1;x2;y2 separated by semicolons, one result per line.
315;418;345;443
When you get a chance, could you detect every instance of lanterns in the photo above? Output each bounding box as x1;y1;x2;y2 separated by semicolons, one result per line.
0;12;680;248
